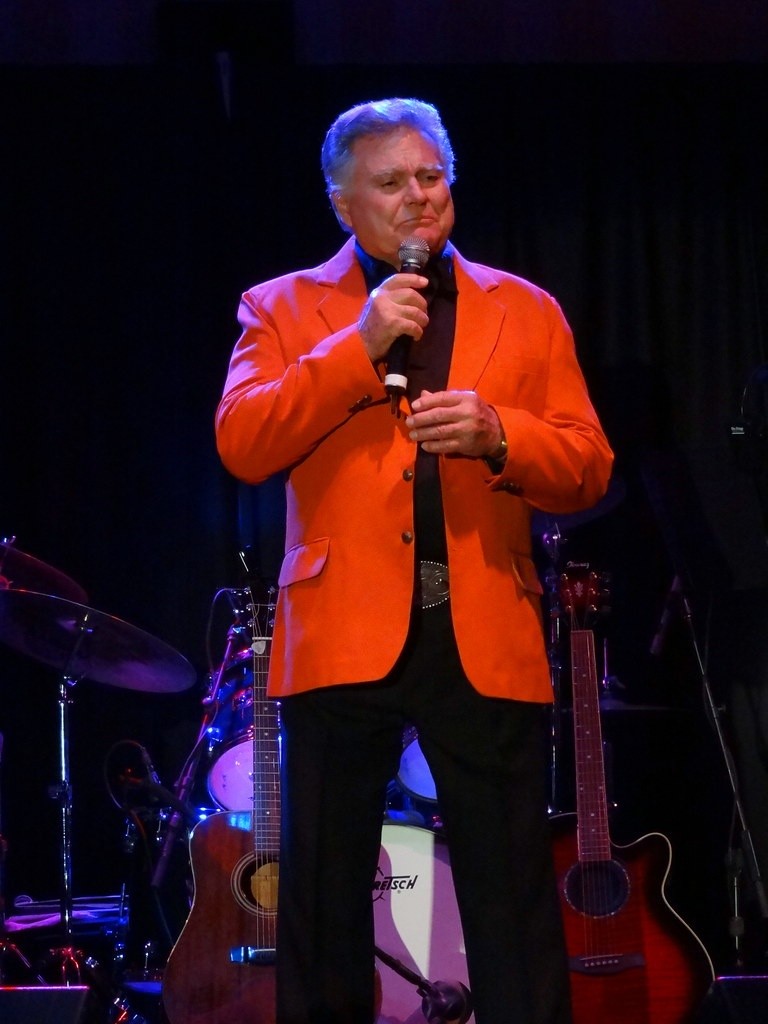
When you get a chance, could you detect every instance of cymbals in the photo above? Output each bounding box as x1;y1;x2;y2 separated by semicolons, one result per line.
559;685;695;715
2;540;92;605
1;584;201;694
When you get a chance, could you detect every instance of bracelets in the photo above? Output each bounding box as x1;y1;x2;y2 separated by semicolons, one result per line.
490;434;507;458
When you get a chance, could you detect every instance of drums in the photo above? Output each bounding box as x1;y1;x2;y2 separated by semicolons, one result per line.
396;724;444;802
204;647;282;813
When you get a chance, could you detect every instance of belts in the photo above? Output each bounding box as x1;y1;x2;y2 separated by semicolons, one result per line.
411;559;450;609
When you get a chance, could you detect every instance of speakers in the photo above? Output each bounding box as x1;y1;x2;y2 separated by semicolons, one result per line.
0;984;108;1024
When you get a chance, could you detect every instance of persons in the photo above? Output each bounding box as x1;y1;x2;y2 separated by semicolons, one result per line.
214;98;615;1024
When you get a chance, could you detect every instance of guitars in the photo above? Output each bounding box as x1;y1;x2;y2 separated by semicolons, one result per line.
163;532;431;1023
542;559;719;1023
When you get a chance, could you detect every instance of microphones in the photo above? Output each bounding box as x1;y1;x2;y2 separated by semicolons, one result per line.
423;982;465;1021
384;237;430;414
140;747;167;804
226;591;254;648
650;573;682;655
